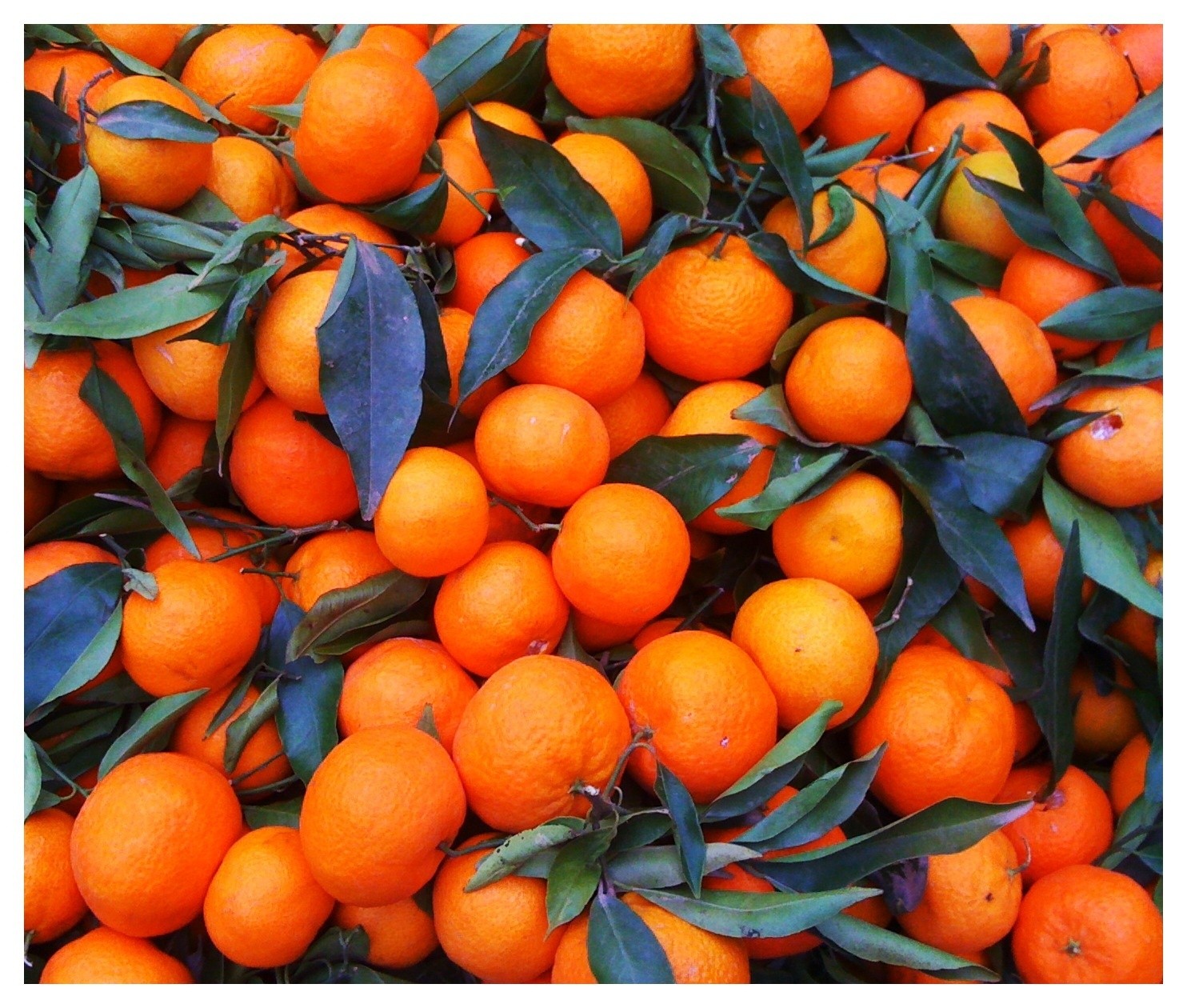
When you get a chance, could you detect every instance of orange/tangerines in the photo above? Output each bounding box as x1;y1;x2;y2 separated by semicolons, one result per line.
24;24;1163;984
